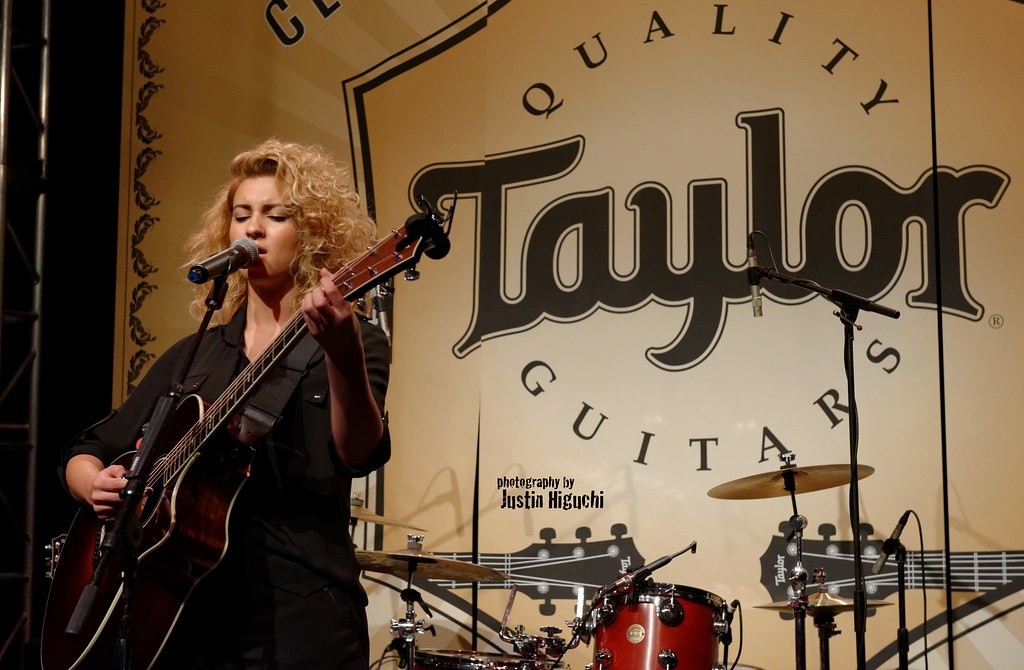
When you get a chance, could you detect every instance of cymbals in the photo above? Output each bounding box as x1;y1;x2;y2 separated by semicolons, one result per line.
349;504;428;533
706;463;876;501
353;546;511;584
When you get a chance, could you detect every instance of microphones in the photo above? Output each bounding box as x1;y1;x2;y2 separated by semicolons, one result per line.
605;541;697;594
188;238;259;285
720;601;738;646
747;234;762;318
385;626;436;654
872;512;910;575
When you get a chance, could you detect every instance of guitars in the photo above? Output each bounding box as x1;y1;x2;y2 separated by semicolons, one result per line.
40;191;450;670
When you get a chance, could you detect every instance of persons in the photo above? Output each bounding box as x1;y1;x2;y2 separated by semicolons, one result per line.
62;138;391;670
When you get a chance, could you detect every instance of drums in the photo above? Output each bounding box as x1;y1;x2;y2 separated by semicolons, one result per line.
591;580;727;670
417;649;567;670
